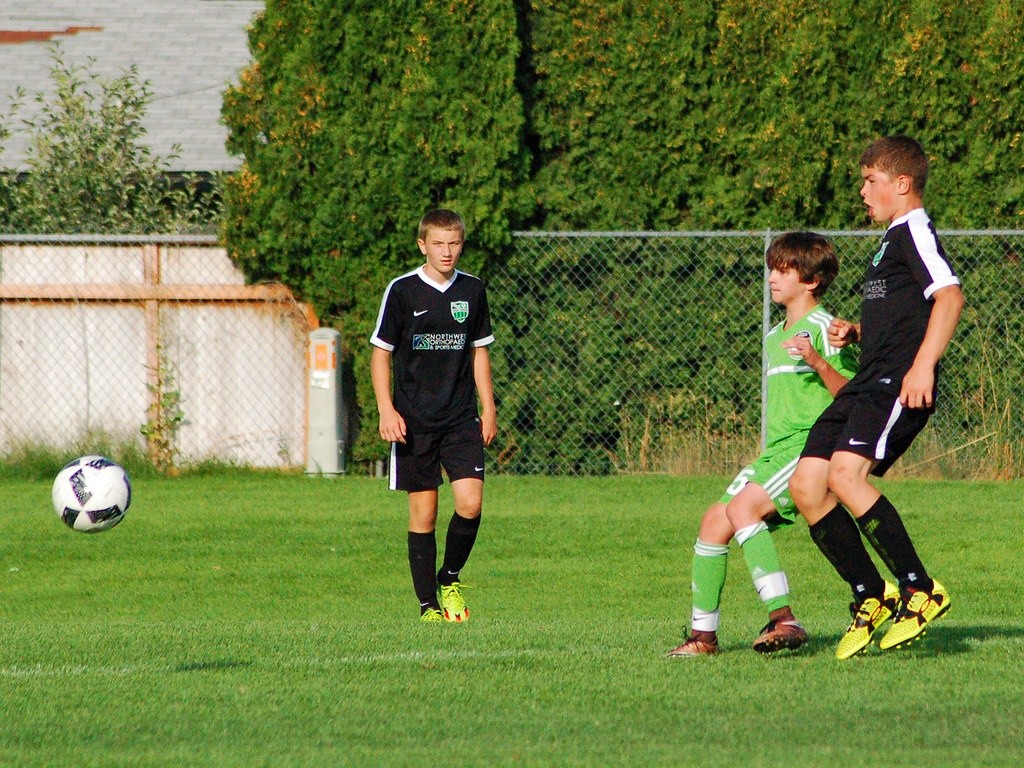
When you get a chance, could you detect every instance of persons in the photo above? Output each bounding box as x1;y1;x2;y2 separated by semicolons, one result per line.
369;209;498;624
662;231;862;659
787;135;966;659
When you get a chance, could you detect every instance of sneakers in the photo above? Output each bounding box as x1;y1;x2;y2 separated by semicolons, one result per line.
667;625;719;657
436;581;473;623
879;578;951;650
419;607;446;626
753;618;810;656
835;579;901;660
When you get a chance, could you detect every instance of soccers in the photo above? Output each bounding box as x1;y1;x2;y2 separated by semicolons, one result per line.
52;455;131;534
